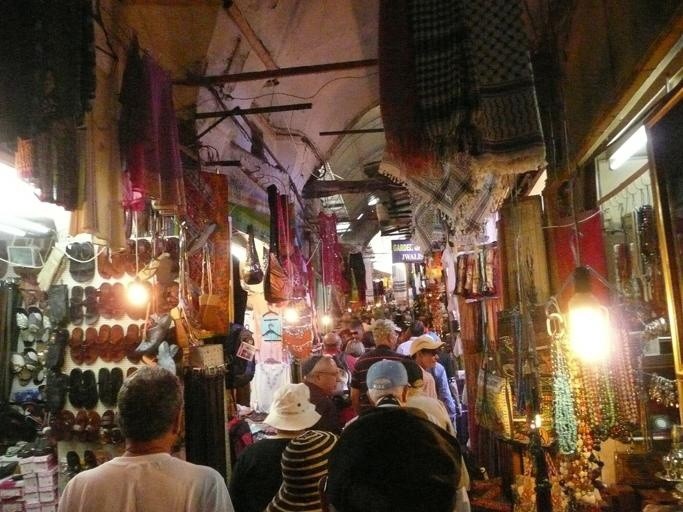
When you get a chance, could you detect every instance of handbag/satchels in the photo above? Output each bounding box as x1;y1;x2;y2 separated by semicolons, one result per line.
279;308;314;362
242;247;264;285
474;368;514;442
264;243;314;305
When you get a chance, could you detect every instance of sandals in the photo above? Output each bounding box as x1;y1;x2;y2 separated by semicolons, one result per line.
124;324;140;364
84;410;101;444
83;286;100;326
81;327;98;365
109;325;124;363
73;410;88;443
98;367;122;408
83;450;97;470
81;368;99;409
66;451;81;479
69;286;84;326
65;241;81;282
134;313;184;375
97;242;113;279
95;325;111;363
77;241;95;283
68;367;83;410
110;242;126;278
126;240;152;278
99;410;125;448
51;411;74;442
68;327;83;365
99;282;127;320
154;281;179;317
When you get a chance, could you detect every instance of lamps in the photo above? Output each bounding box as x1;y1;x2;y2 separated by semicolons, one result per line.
566;266;600;322
610;126;647;172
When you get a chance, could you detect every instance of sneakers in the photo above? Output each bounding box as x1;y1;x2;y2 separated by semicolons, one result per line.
9;348;47;387
14;306;51;348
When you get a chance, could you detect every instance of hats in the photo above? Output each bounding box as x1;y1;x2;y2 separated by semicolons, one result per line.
409;335;447;357
366;358;411;389
372;317;403;334
262;382;322;432
301;355;322;376
400;360;424;388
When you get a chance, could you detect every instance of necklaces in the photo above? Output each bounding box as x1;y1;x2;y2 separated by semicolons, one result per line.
550;325;640;454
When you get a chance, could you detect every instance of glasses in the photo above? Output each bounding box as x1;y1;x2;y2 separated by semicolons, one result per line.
317;369;340;377
422;350;441;356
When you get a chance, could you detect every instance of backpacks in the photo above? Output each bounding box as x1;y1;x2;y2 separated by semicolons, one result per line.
224;323;256;389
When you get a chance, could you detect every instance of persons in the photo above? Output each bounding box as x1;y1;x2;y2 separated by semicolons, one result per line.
57;367;234;512
228;382;322;512
302;301;472;512
